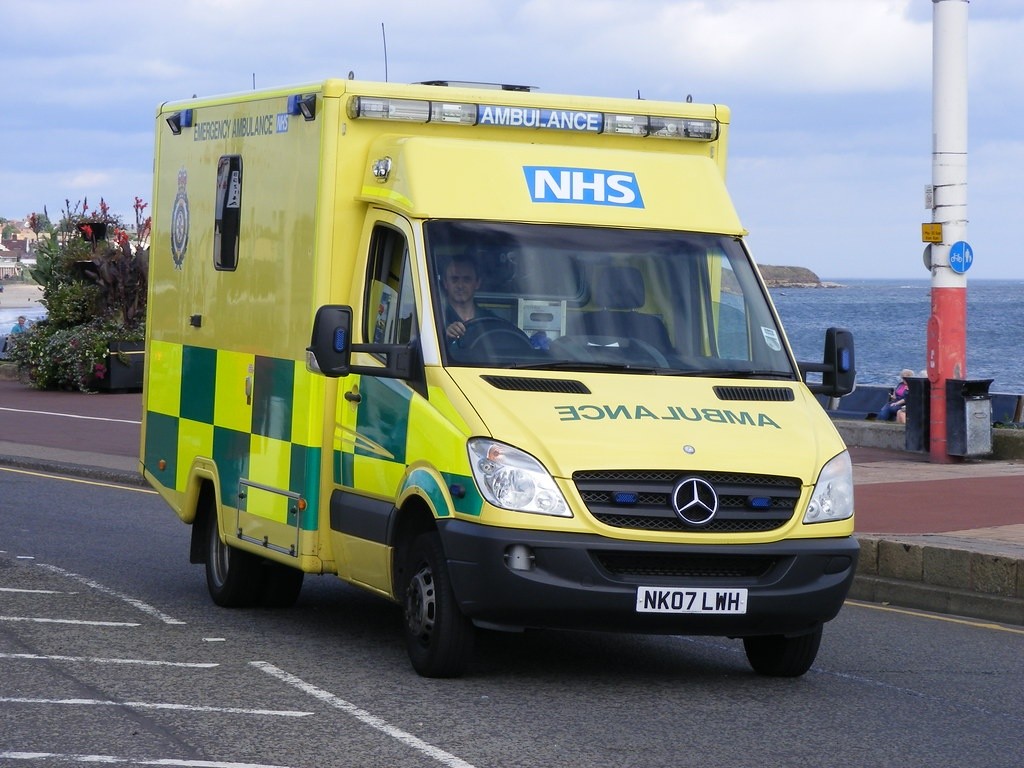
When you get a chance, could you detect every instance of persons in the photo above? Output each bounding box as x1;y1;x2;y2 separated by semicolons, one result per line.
877;369;915;421
11;316;28;335
896;369;929;423
411;254;527;358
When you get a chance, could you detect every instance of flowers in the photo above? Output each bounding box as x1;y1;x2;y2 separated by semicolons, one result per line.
4;196;152;395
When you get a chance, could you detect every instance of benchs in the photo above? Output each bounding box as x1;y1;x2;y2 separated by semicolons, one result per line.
806;382;1019;426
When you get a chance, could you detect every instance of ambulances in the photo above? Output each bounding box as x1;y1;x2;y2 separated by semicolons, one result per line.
137;79;860;679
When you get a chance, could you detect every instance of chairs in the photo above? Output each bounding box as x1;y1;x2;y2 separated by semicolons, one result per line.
570;265;677;356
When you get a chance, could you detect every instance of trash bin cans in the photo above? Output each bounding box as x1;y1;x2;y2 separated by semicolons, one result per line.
944;377;996;459
902;375;931;453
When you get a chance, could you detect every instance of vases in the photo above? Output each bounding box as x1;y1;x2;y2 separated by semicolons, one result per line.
88;341;145;394
